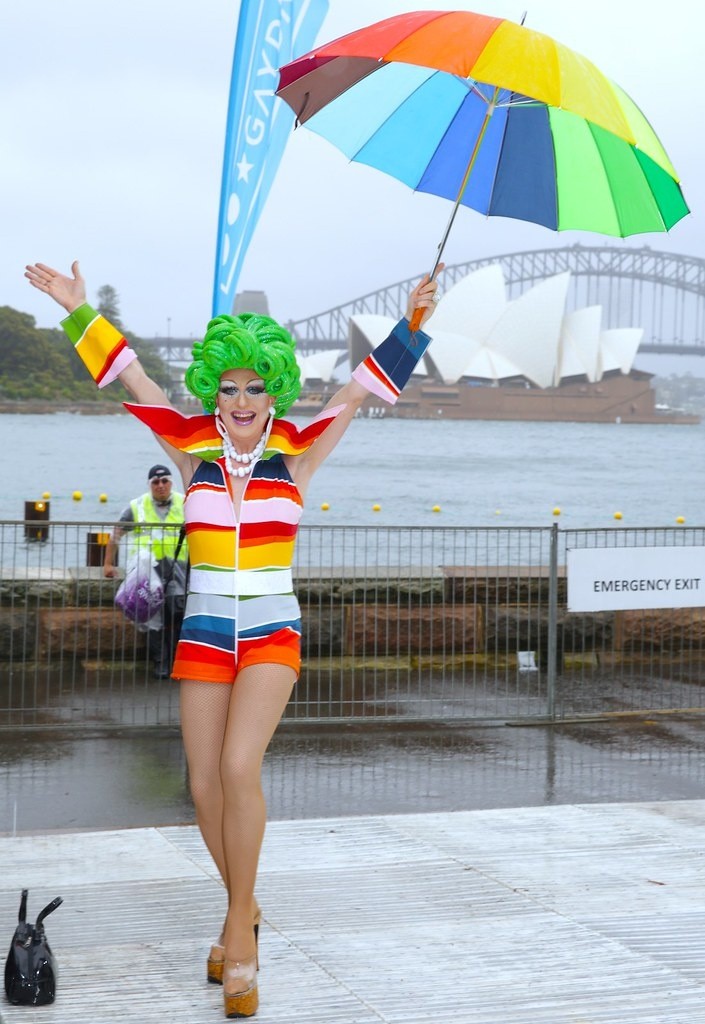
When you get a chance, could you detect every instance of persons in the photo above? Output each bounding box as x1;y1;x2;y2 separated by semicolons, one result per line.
22;261;445;1020
103;464;191;680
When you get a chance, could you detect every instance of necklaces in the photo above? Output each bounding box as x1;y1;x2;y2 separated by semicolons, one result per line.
221;430;267;478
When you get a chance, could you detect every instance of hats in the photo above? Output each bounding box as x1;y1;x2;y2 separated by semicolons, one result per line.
147;464;170;480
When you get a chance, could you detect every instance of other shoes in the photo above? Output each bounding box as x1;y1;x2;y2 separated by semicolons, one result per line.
154;660;168;679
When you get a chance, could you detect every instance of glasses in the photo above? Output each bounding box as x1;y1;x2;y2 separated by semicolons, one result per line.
152;479;167;485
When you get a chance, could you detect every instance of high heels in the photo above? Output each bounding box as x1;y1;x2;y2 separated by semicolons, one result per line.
207;908;260;984
222;954;257;1018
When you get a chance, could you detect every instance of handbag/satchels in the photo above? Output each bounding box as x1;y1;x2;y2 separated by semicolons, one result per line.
4;889;63;1005
112;549;163;632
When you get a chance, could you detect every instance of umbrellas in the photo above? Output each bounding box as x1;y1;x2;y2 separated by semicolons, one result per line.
271;9;690;348
